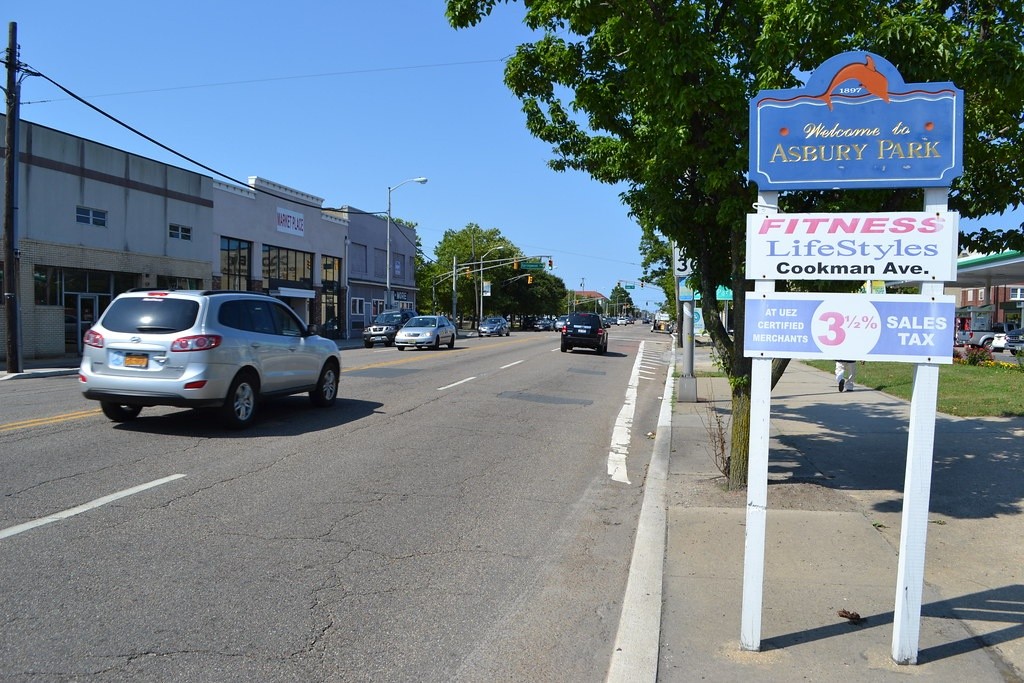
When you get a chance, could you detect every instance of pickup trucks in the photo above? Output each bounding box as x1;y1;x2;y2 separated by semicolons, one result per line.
957;323;1018;353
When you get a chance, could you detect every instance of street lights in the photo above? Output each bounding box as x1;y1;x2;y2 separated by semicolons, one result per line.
479;246;505;324
385;177;428;309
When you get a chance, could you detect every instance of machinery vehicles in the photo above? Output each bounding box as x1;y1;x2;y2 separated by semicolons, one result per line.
650;310;674;334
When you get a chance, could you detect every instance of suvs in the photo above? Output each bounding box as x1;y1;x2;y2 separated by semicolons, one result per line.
361;308;419;348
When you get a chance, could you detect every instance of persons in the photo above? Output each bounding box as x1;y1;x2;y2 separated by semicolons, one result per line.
834;360;857;392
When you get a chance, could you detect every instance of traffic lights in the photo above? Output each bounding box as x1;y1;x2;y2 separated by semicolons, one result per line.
618;282;620;286
528;276;532;284
514;260;520;270
641;283;643;288
548;259;553;270
466;269;470;278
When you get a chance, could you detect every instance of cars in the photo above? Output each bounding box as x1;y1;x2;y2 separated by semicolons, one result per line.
523;316;568;332
991;334;1009;349
77;287;342;429
646;316;652;322
603;317;634;328
560;312;611;354
477;318;510;337
642;318;649;324
1004;326;1024;357
394;315;456;351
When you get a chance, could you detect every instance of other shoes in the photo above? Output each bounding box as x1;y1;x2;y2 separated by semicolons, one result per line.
839;379;844;391
